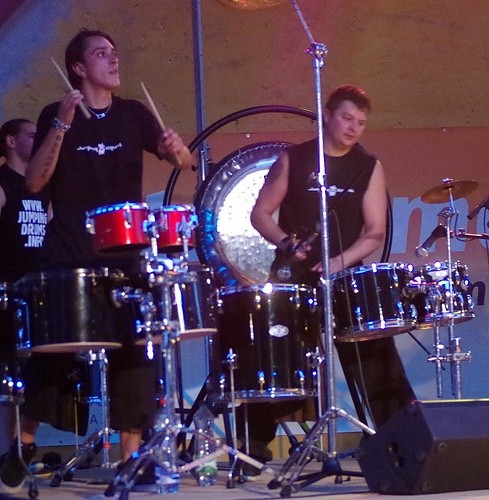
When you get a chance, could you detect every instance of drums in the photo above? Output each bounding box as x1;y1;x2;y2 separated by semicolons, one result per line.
327;261;421;343
210;282;323;406
0;281;30;405
414;260;476;330
132;259;221;346
18;268;135;354
85;201;155;256
151;204;199;253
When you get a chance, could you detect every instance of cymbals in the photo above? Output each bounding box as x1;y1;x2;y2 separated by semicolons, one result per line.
420;180;480;204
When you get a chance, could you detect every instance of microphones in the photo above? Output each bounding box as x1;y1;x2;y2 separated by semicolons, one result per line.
467;195;489;221
414;224;444;260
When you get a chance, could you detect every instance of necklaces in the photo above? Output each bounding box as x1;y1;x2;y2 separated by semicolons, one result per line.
85;102;111;118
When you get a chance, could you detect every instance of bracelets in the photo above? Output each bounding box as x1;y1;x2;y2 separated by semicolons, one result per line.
52;117;71;132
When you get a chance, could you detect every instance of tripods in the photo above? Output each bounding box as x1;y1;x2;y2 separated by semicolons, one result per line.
265;0;380;499
100;261;279;497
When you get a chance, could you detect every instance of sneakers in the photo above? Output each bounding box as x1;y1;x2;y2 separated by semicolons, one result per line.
0;437;37;495
115;454;162;492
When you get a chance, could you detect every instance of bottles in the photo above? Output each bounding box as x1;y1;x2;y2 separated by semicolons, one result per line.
153;399;181;494
193;401;218;487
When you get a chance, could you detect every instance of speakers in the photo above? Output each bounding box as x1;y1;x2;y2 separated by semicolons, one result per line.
356;400;489;494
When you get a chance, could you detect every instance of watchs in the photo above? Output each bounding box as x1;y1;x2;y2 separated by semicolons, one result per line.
278;237;290;250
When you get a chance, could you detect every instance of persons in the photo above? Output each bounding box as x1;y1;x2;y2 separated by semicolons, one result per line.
0;30;194;493
241;84;387;479
0;120;52;286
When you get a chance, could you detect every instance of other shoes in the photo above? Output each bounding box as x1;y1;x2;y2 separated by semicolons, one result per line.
238;452;265;483
